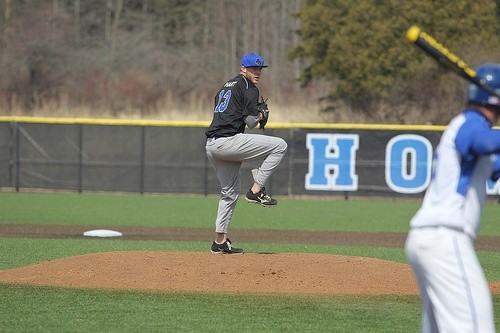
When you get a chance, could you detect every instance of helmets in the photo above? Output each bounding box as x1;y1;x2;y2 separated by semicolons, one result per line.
467;64;500;105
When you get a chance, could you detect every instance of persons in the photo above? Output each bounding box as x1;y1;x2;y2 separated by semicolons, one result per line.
205;52;287;253
405;63;500;333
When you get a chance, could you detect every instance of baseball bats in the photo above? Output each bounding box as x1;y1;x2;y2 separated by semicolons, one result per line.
404;25;500;100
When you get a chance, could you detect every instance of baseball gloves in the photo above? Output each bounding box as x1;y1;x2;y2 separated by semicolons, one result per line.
257;96;270;131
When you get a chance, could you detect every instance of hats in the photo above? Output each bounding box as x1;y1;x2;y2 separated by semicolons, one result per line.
242;53;269;68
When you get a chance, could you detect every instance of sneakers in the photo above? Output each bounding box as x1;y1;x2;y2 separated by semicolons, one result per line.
245;187;277;208
210;238;243;254
